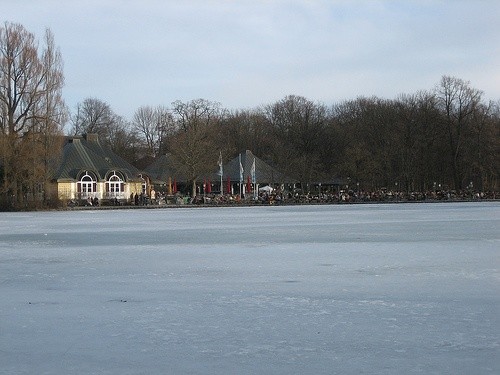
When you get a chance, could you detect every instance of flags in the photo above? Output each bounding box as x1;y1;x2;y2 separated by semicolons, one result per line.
216;157;224;176
239;156;246;182
250;157;256;183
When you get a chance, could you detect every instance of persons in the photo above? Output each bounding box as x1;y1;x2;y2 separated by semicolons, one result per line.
82;184;500;207
